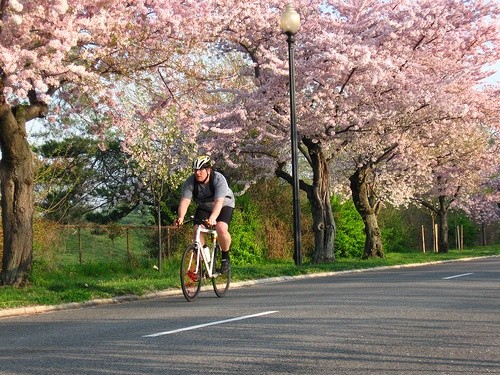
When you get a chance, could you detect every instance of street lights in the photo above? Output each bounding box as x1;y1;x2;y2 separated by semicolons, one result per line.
279;1;302;266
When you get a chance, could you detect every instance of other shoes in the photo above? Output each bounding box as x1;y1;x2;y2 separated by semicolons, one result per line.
220;258;232;274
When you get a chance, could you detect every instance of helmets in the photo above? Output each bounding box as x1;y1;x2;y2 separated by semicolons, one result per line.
192;156;212;170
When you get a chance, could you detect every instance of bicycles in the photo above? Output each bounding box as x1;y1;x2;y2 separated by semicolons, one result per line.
180;215;232;302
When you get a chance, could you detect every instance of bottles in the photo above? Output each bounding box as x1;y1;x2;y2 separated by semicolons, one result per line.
203;244;211;263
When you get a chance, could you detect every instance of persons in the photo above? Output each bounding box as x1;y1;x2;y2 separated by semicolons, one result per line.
174;155;235;274
